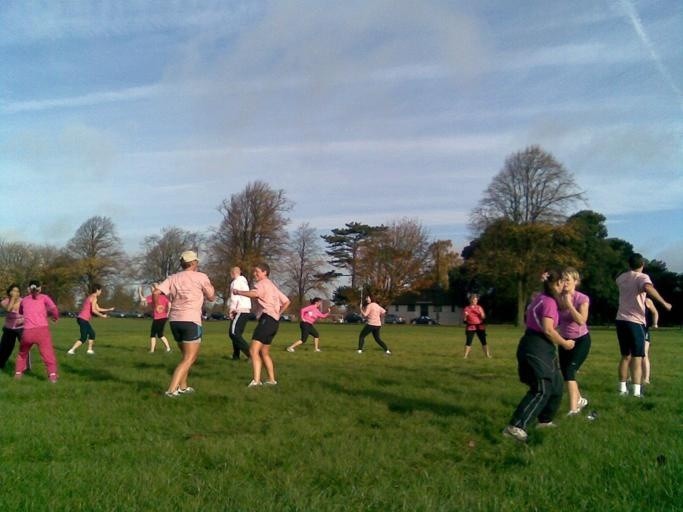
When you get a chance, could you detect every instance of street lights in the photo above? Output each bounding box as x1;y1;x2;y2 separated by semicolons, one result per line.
358;287;364;313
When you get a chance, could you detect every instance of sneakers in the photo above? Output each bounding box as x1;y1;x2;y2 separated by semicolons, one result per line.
357;350;361;353
315;349;321;352
15;374;21;379
87;350;94;353
502;425;527;441
49;375;57;383
286;348;294;352
618;391;645;398
165;387;195;398
68;350;74;354
567;398;588;415
248;379;277;387
537;422;556;428
386;350;390;353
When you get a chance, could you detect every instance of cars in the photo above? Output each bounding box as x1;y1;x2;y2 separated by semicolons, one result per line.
201;314;207;320
65;312;76;317
410;316;439;325
385;314;406;324
127;310;141;318
280;315;292;321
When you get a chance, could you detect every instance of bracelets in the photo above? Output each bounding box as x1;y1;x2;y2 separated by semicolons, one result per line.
235;290;238;295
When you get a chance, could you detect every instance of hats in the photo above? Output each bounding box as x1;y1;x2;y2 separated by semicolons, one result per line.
180;251;199;262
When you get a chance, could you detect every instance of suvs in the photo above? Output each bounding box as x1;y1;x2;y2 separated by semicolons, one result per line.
108;310;126;317
211;312;227;320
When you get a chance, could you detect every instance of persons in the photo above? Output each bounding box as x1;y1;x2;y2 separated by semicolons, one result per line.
0;283;30;372
503;253;672;440
136;282;171;354
461;294;491;359
65;283;114;355
232;261;292;387
354;294;392;355
14;280;59;383
285;297;331;352
151;250;216;397
227;266;252;361
